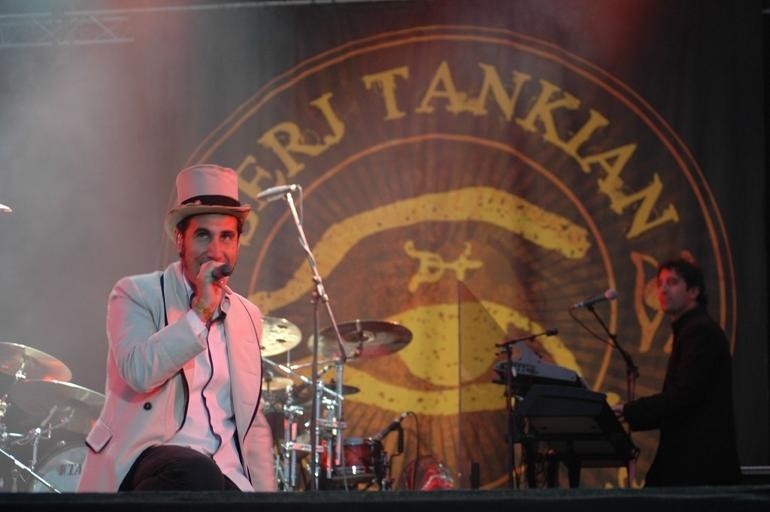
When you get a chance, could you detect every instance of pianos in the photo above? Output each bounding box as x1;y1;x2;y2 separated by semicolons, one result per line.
492;342;640;468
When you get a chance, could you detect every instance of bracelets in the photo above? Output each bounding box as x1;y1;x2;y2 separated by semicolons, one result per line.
191;294;218;321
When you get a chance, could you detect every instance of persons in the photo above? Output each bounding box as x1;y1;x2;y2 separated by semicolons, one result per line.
611;255;744;487
78;162;276;491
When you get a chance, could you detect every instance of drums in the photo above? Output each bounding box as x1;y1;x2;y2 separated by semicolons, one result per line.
319;438;387;481
22;442;89;493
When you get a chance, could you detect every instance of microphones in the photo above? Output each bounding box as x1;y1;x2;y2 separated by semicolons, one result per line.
256;183;297;201
366;412;409;449
545;328;559;336
211;263;232;281
574;288;618;309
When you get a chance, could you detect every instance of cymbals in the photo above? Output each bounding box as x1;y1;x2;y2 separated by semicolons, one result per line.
0;342;72;382
308;320;413;358
10;379;106;434
261;378;294;390
260;316;302;357
295;382;359;400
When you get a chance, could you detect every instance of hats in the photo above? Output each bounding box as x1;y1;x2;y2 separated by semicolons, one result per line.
163;164;251;244
